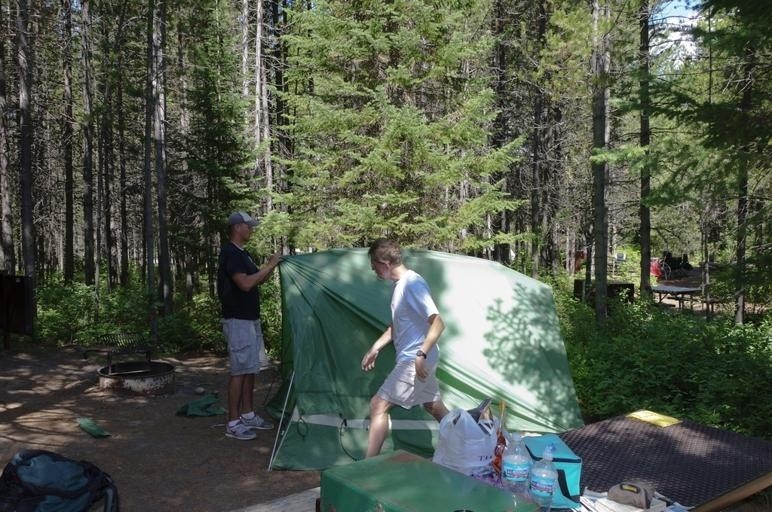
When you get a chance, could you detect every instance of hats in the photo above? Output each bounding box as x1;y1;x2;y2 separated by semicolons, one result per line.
229;211;261;226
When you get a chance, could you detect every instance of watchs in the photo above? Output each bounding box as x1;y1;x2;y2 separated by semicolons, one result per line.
416;350;426;359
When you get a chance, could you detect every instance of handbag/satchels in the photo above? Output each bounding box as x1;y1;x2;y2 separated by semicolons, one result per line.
522;433;583;498
1;448;119;511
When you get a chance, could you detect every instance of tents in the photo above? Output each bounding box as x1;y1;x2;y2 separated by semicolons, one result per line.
268;247;585;471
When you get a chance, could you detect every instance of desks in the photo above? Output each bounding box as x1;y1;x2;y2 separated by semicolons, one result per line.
557;407;770;512
643;286;700;310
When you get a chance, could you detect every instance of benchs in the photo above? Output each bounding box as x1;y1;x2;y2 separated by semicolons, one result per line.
665;297;723;313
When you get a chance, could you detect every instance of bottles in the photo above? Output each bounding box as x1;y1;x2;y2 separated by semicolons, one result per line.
501;431;532;502
528;447;558;512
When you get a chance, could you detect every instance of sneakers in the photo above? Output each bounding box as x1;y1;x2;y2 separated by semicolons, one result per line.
225;410;275;440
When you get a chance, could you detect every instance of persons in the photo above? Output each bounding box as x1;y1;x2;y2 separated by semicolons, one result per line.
217;212;285;441
361;238;450;458
